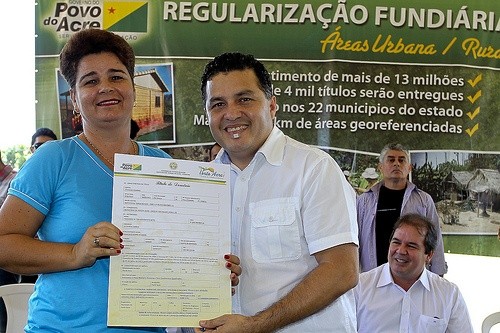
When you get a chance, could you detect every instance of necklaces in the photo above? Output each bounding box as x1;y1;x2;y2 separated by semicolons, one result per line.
84;136;138;168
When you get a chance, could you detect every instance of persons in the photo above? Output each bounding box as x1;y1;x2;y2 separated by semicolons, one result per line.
353;214;472;333
194;51;362;333
0;28;244;333
356;142;447;279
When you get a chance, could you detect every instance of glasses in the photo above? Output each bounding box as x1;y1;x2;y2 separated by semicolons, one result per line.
32;142;45;149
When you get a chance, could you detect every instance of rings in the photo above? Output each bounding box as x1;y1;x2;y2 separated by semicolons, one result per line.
94;237;100;247
